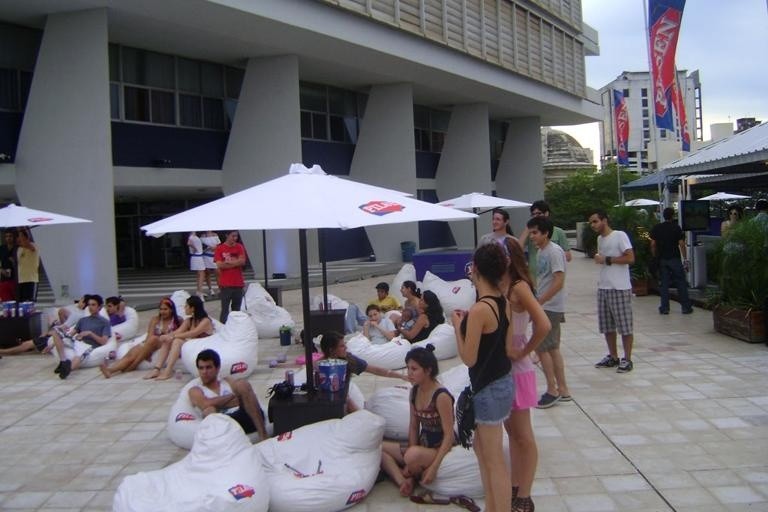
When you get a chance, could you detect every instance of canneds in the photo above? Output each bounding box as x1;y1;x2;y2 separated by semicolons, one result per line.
328;299;332;311
284;369;294;392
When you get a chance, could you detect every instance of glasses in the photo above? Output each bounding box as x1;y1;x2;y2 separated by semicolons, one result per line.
729;211;737;216
531;212;544;216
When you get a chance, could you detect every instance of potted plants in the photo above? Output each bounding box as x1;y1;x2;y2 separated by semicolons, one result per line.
697;209;768;344
609;199;649;296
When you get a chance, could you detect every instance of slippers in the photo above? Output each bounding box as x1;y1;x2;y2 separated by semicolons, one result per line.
409;491;452;506
556;394;572;401
534;392;562;409
450;493;481;512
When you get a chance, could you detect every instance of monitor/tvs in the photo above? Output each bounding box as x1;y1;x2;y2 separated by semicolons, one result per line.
681;199;712;231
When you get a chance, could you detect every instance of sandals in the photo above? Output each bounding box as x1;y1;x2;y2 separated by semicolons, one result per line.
141;367;160;380
155;369;176;381
511;486;535;511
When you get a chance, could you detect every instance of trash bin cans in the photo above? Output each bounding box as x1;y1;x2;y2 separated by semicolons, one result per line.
400;241;416;263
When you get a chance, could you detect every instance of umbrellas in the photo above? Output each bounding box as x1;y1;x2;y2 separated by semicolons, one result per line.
613;198;664;208
0;203;93;281
312;162;414;315
136;160;483;398
697;192;752;202
431;190;533;250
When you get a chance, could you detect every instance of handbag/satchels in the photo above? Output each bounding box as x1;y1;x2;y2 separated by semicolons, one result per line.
455;387;477;449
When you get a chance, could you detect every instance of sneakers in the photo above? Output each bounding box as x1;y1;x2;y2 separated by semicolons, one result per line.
680;307;694;315
616;358;633;374
657;306;671;315
594;354;621;369
195;290;208;302
59;358;72;379
208;288;216;297
52;360;66;375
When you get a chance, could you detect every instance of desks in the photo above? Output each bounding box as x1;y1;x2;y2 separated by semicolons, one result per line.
0;314;42;348
268;388;348;436
309;310;347;341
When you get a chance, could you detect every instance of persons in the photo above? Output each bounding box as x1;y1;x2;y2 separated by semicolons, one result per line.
0;227;247;380
187;348;269;442
647;206;694;315
451;197;572;512
361;282;447;343
749;199;768;222
319;329;411;414
586;207;636;373
720;203;743;236
377;343;455;497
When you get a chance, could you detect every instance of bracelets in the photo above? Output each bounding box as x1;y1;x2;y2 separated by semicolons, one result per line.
605;255;612;266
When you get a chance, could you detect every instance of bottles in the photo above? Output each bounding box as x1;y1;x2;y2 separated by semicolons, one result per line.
280;325;291;345
11;308;16;317
18;307;24;317
313;370;319;390
285;370;293;386
